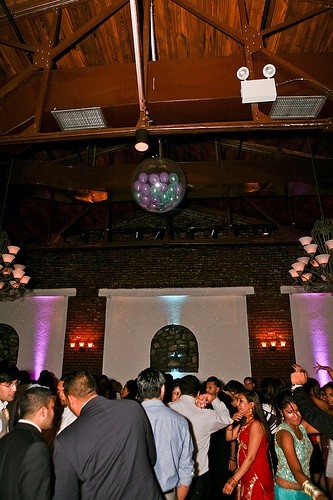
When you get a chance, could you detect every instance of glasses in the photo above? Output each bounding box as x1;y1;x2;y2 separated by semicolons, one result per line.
1;380;21;389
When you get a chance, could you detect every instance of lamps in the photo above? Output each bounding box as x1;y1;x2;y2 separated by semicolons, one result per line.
135;231;143;240
50;106;108;133
185;229;195;240
268;96;328;119
154;231;165;240
210;229;218;238
134;129;150;152
95;230;105;235
0;158;33;303
287;135;333;293
236;63;278;104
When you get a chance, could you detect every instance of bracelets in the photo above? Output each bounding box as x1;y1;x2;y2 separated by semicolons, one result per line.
230;455;237;462
228;476;238;485
301;478;322;499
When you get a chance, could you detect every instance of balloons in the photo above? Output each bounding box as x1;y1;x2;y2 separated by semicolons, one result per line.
132;173;188;213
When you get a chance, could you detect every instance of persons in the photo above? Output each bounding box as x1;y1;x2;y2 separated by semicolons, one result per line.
0;354;333;500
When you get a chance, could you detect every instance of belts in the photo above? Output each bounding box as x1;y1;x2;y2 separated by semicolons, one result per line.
163;489;174;494
